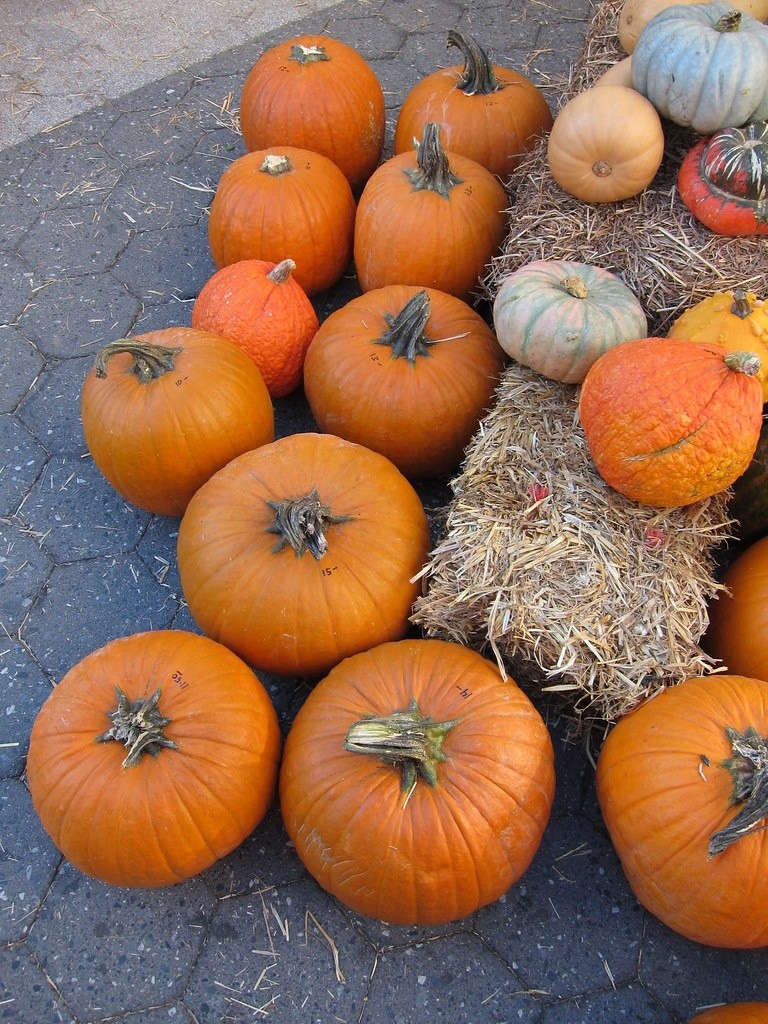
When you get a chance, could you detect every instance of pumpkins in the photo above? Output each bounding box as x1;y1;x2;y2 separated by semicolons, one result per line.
27;0;768;1024
